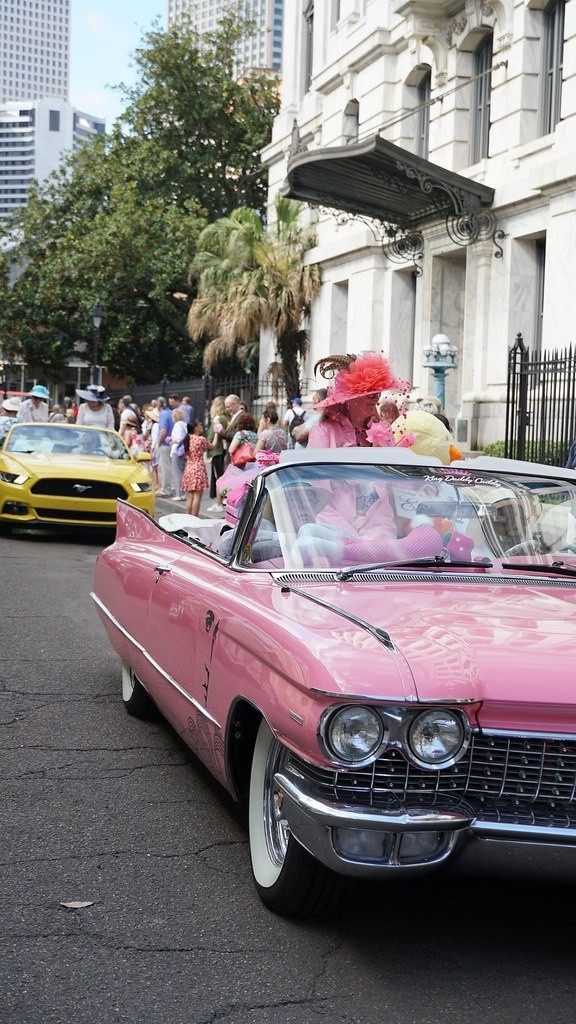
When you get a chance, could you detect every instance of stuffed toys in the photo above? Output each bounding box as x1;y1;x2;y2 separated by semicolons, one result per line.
388;411;463;546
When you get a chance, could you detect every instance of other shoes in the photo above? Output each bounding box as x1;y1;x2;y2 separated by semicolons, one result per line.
172;496;183;501
207;504;224;512
181;495;187;500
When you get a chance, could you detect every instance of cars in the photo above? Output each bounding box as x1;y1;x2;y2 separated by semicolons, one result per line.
90;444;576;920
0;422;156;537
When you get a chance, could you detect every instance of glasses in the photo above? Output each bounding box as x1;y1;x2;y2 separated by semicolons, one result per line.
288;497;319;507
241;408;243;410
365;392;381;399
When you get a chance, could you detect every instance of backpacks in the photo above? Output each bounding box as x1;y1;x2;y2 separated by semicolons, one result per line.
289;409;306;438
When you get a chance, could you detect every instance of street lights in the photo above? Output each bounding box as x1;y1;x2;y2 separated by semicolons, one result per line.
420;334;457;412
91;302;105;386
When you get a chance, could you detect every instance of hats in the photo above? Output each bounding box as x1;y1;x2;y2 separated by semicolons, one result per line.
146;408;159;423
466;488;542;543
308;354;412;410
27;385;53;401
291;398;302;405
75;385;111;401
127;415;137;426
262;482;330;522
2;397;22;411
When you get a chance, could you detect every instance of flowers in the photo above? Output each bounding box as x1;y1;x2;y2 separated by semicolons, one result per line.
366;421;392;448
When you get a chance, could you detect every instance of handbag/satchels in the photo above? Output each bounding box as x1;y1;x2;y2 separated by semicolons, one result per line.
232;431;255;468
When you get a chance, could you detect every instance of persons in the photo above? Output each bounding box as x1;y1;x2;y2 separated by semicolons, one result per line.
182;415;220;517
0;397;21;417
219;479;329;565
117;394;194;500
228;413;258;471
253;402;289;461
381;403;399;424
16;386;50;423
417;397;450;431
292;388;328;449
71;434;127;459
50;396;77;421
206;394;248;512
75;385;115;429
304;353;411;538
283;398;310;450
